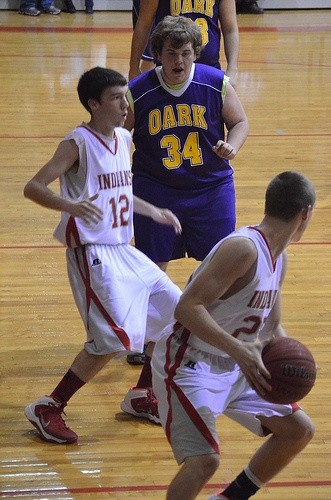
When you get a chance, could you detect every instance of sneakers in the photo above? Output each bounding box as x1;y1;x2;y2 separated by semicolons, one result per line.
19;6;41;16
120;386;162;426
36;6;61;15
24;394;78;445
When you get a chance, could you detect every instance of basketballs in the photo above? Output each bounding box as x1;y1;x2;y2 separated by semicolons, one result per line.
252;336;316;405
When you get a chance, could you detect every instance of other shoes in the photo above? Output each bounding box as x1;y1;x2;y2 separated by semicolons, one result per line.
68;7;76;13
85;7;93;13
243;3;263;14
127;345;148;366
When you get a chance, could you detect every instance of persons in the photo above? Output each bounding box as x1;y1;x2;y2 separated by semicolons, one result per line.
235;0;264;15
24;67;183;444
128;0;240;92
19;0;62;16
122;15;248;366
64;0;94;13
151;172;314;500
